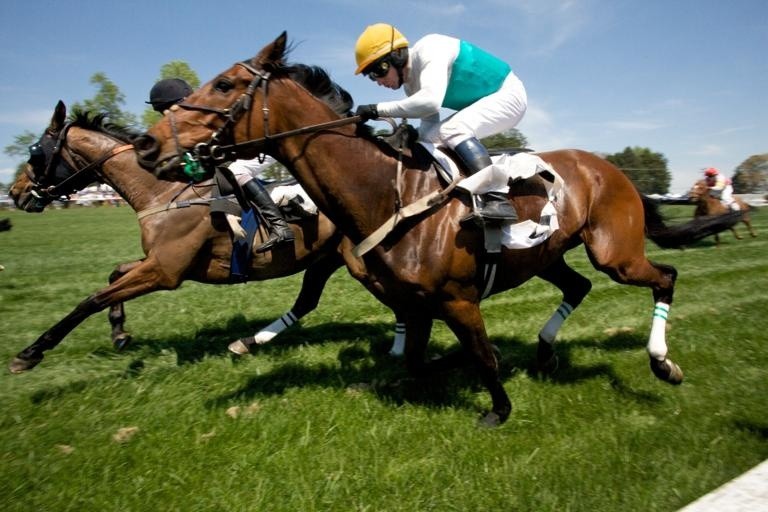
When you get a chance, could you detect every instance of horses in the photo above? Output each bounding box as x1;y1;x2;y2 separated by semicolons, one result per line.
685;177;759;244
5;97;408;376
130;28;759;430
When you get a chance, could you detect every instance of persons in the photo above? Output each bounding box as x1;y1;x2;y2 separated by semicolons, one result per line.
143;77;296;254
694;167;741;216
352;24;527;231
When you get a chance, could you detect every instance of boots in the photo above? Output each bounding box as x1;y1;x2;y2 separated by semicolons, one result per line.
239;179;296;253
455;136;520;232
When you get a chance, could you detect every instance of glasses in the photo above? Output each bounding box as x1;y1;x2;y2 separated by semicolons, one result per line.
360;62;391;81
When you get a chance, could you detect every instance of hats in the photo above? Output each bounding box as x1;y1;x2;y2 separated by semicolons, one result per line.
143;76;193;105
703;166;720;180
352;23;408;77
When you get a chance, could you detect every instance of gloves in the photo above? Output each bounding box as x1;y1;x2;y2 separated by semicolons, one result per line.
393;125;421;153
350;101;381;127
